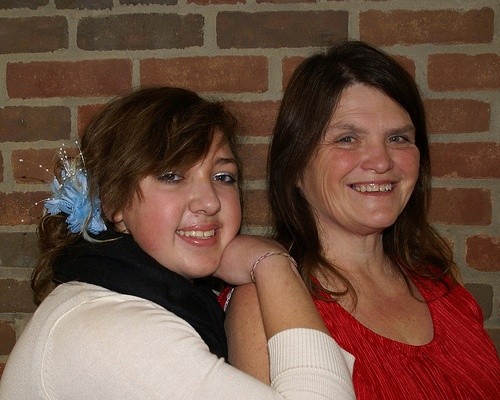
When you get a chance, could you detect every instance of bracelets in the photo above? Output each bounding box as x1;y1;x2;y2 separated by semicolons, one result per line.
250;250;298;284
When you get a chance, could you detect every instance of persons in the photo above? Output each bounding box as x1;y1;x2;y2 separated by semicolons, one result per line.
218;41;500;399
0;86;356;400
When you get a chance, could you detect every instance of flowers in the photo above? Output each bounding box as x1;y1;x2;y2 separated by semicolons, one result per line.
22;140;109;235
